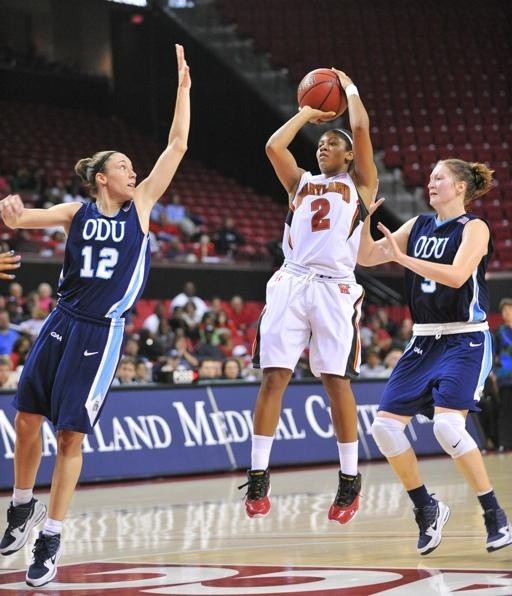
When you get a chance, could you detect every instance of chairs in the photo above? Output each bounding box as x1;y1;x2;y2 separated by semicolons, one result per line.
0;0;512;355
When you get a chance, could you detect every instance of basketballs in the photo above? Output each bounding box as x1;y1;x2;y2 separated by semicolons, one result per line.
297;68;347;121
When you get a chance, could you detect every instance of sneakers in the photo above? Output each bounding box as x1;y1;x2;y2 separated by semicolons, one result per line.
27;532;62;587
328;471;362;526
481;509;511;554
243;468;273;519
1;498;48;557
411;501;451;556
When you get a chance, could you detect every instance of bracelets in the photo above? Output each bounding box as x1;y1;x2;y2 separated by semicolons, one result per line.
343;83;361;98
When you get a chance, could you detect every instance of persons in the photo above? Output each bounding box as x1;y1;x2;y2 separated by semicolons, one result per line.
0;38;192;588
1;165;88;258
152;194;216;264
171;281;206;323
356;157;512;557
237;66;381;526
286;297;512;455
1;283;56;392
110;298;262;384
216;217;254;261
